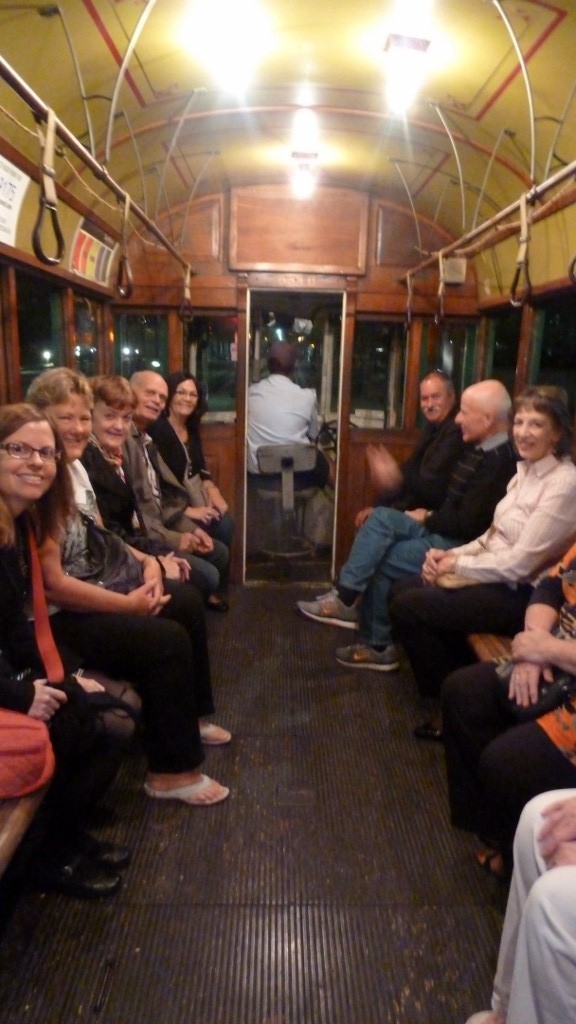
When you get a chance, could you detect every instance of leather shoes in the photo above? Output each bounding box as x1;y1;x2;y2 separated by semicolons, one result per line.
39;838;131;899
414;715;443;739
208;599;229;612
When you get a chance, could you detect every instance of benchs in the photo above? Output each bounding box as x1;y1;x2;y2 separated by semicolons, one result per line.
0;757;56;893
463;633;576;789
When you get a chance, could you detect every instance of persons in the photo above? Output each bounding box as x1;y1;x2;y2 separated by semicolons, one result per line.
297;373;575;1024
1;366;235;904
248;339;336;492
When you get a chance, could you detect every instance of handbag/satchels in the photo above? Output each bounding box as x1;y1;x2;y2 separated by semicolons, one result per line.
183;473;209;507
0;706;56;800
500;653;574;721
62;523;145;594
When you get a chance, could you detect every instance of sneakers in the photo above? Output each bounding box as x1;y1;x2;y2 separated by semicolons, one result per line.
297;588;360;628
334;640;401;671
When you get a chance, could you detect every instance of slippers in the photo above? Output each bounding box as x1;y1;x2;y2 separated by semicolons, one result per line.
144;772;229;804
199;721;231;743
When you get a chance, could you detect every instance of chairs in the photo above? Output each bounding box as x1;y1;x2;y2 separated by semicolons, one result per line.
252;444;328;572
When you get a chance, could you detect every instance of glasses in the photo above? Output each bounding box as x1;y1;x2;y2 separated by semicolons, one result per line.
0;440;63;462
